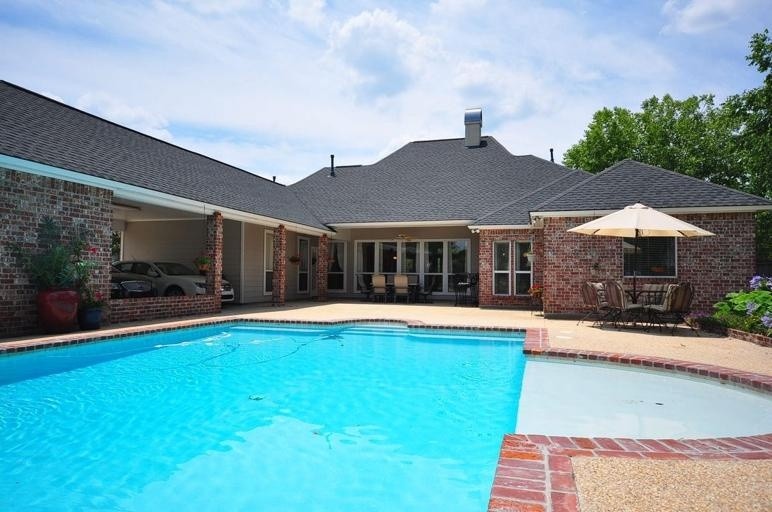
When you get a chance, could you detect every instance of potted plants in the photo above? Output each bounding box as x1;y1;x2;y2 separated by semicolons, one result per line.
6;212;92;330
527;250;536;261
195;254;210;270
289;255;300;264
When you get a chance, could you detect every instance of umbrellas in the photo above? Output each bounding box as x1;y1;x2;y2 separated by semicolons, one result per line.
566;201;717;305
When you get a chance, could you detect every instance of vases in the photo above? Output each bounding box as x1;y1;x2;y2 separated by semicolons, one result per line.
80;307;101;330
532;292;541;301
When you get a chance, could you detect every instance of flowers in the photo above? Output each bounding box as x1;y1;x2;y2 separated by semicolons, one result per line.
70;228;110;311
527;283;543;293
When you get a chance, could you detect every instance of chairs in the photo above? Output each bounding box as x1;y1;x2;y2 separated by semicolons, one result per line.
576;278;699;336
355;274;436;305
453;273;479;307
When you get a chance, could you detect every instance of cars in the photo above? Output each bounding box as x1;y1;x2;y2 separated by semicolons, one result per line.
111;261;234;304
